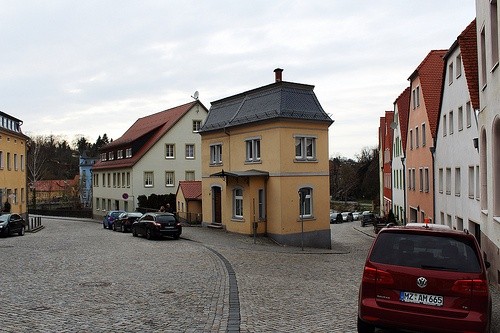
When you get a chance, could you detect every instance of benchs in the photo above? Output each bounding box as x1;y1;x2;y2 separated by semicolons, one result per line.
389;240;460;269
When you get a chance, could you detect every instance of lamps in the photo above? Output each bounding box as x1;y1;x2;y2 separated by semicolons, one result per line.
473;138;478;148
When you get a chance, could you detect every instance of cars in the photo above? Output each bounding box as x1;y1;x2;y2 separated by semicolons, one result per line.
357;222;492;333
103;210;126;230
111;212;144;233
0;212;27;238
330;210;392;234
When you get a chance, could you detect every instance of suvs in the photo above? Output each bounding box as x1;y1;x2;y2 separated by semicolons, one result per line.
132;211;182;240
